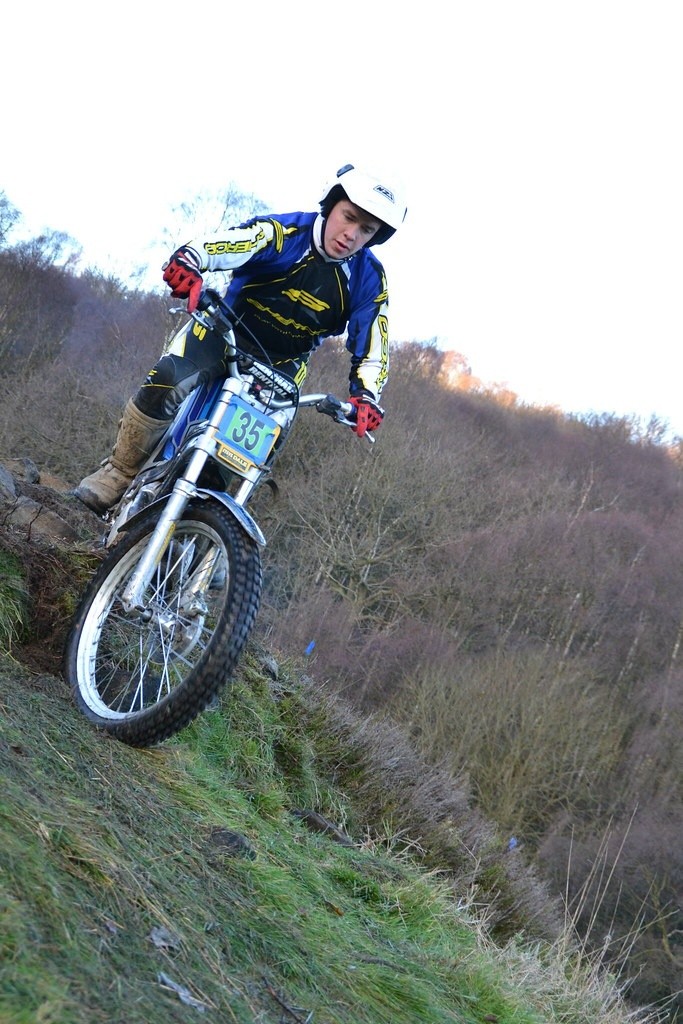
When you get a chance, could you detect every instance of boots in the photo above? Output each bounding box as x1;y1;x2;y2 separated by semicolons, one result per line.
72;395;174;516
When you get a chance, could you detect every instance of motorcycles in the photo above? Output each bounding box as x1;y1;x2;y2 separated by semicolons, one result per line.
62;261;376;750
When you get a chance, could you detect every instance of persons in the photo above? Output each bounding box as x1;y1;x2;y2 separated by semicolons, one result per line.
72;161;408;587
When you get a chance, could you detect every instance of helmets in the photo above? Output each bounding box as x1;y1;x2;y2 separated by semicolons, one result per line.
318;159;408;249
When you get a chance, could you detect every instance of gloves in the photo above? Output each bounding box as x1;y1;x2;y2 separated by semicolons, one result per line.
346;394;386;438
163;245;204;314
